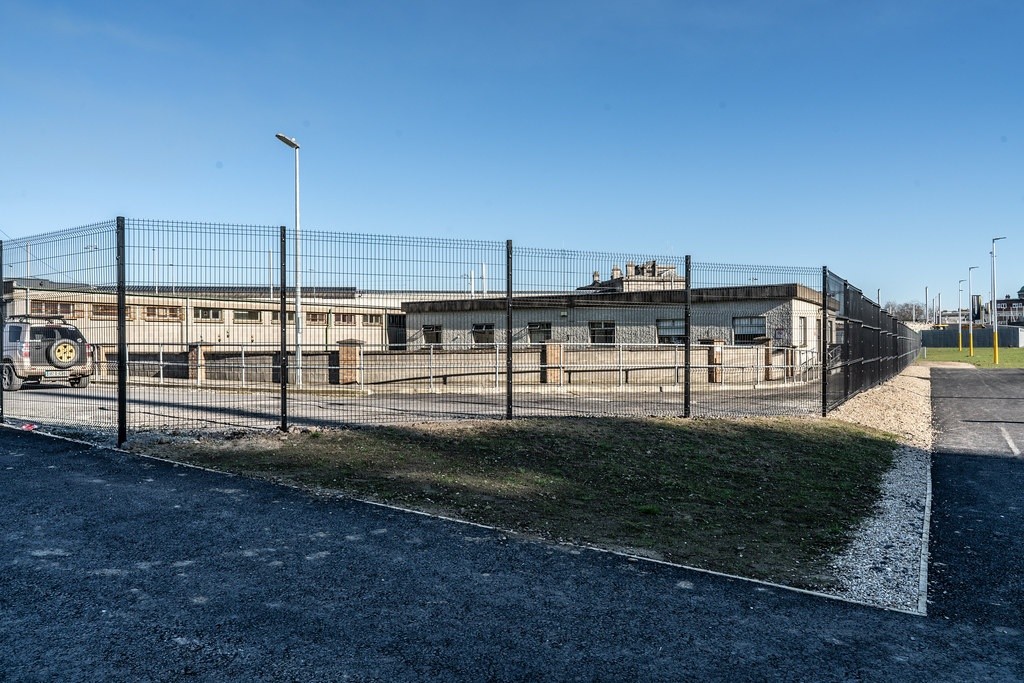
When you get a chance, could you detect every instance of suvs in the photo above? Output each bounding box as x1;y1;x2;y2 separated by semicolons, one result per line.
2;315;93;391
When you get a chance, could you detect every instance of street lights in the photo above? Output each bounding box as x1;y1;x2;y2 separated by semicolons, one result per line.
993;236;1007;364
275;132;302;385
959;280;968;352
969;266;978;357
